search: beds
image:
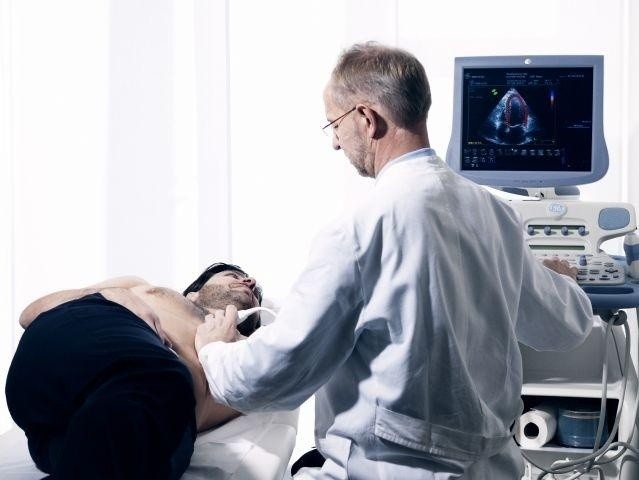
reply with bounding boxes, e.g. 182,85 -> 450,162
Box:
0,410 -> 300,480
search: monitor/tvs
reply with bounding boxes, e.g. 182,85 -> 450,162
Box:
446,55 -> 610,199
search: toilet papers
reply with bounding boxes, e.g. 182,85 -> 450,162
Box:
515,410 -> 557,446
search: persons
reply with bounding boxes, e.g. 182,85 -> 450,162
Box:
193,38 -> 594,480
5,260 -> 264,480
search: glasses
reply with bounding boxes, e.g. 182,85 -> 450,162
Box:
319,108 -> 355,133
